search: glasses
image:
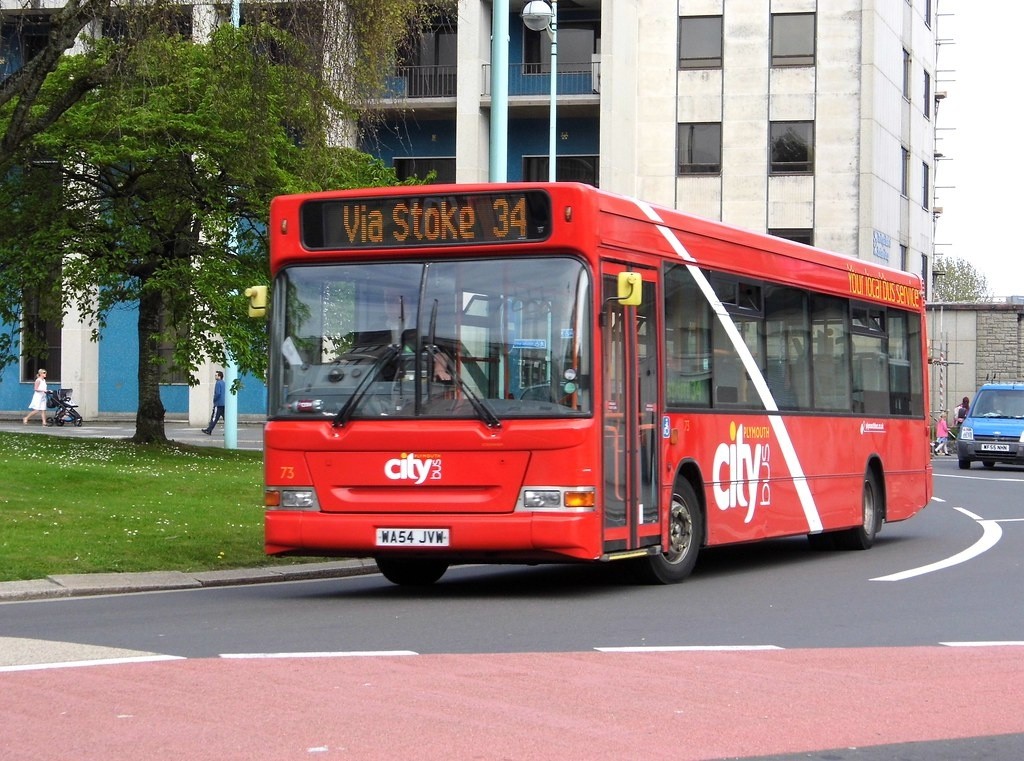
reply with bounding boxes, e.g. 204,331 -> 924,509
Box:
42,372 -> 46,374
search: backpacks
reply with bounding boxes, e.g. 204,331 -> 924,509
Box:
957,407 -> 967,424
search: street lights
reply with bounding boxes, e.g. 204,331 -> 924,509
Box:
521,0 -> 558,184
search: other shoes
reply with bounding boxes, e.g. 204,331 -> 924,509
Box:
202,430 -> 211,436
42,424 -> 48,427
934,450 -> 938,456
23,417 -> 27,425
945,453 -> 951,456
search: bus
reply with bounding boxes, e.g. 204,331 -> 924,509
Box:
242,179 -> 933,586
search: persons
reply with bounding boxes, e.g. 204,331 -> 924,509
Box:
952,396 -> 970,449
354,281 -> 451,384
22,368 -> 52,428
933,412 -> 951,456
201,370 -> 226,435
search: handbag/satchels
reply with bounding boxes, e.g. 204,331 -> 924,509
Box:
47,394 -> 56,408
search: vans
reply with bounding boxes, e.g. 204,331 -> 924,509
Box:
954,382 -> 1024,472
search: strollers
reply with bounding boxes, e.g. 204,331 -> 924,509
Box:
44,388 -> 83,427
